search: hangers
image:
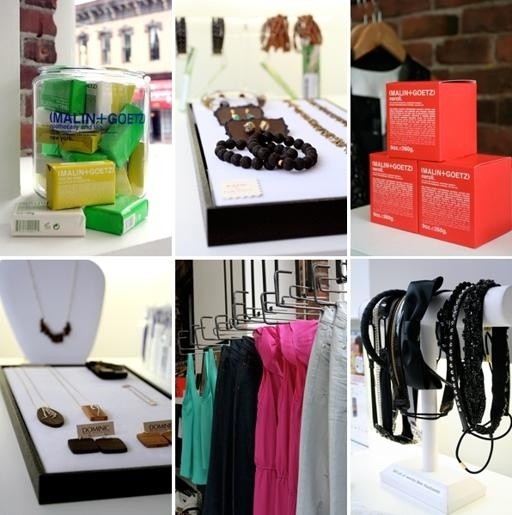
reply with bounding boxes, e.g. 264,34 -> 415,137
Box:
351,0 -> 438,83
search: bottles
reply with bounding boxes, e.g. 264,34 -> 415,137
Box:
30,61 -> 150,198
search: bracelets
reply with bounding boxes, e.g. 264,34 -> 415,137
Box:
246,131 -> 318,170
214,140 -> 298,171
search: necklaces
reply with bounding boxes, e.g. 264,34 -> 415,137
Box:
14,366 -> 64,428
47,366 -> 111,422
28,260 -> 79,345
